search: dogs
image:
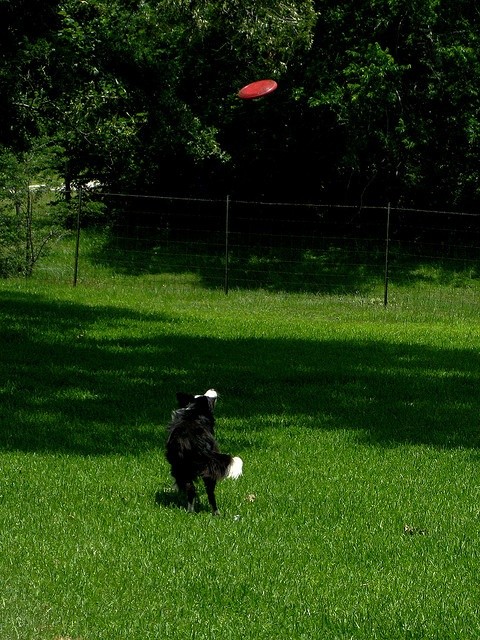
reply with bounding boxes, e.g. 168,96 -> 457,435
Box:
165,389 -> 243,516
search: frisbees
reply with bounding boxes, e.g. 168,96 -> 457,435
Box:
238,80 -> 277,99
